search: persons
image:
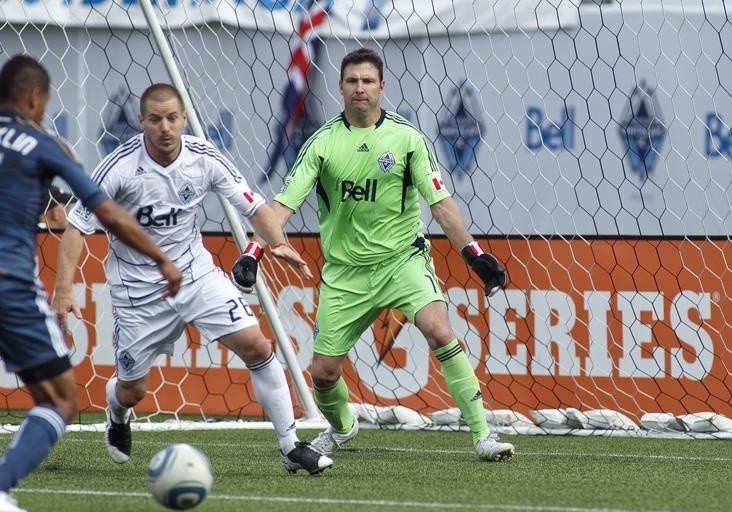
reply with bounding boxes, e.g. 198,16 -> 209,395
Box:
48,82 -> 335,477
228,46 -> 517,463
0,53 -> 184,511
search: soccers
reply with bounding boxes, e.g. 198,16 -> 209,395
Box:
148,442 -> 213,508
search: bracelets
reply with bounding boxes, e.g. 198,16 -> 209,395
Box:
274,242 -> 288,248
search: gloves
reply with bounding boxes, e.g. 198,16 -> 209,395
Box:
460,240 -> 508,297
229,239 -> 264,293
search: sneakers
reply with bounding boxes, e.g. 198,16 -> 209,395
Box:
473,432 -> 517,463
277,440 -> 334,474
102,401 -> 135,465
307,416 -> 360,456
0,491 -> 27,512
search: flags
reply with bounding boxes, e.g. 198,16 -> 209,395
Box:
256,0 -> 329,185
